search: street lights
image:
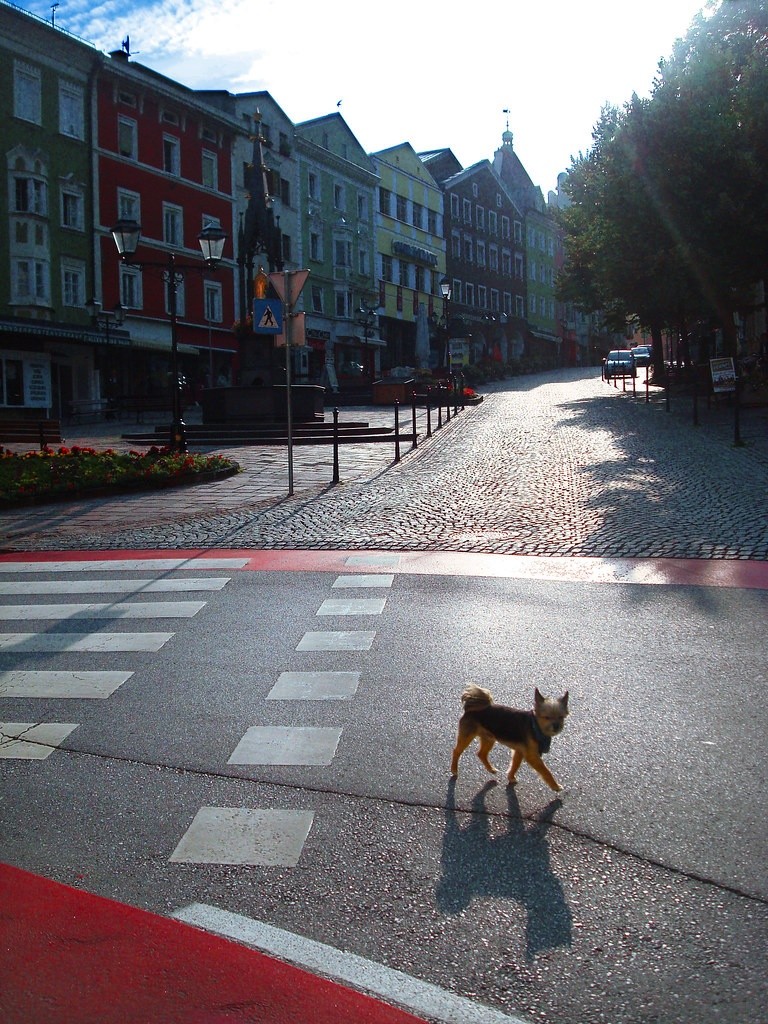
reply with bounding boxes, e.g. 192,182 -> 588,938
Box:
110,210 -> 230,455
440,274 -> 454,387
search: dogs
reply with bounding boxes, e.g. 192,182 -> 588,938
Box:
450,687 -> 570,793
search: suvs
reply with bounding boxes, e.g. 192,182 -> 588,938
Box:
637,345 -> 652,355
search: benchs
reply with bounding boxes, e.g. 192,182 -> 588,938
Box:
64,396 -> 195,425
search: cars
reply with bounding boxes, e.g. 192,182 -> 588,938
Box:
602,350 -> 636,378
631,347 -> 653,367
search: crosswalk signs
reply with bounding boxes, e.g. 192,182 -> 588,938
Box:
252,297 -> 284,334
